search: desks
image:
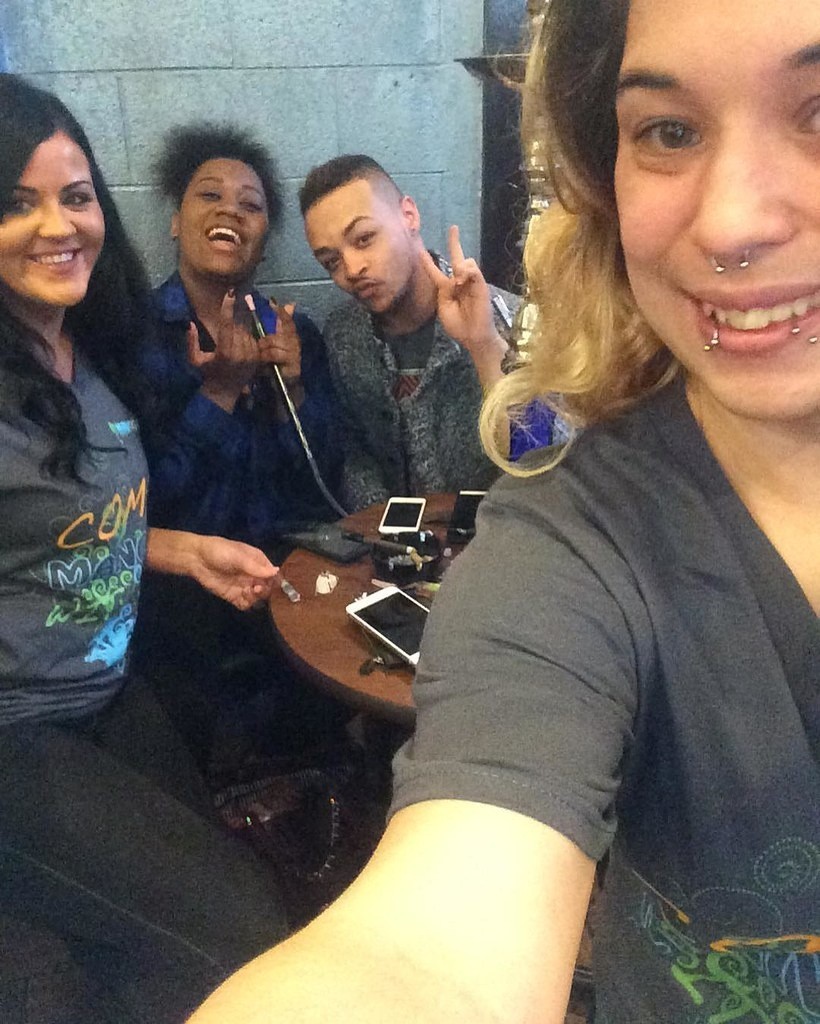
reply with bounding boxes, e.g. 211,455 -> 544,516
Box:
269,493 -> 466,727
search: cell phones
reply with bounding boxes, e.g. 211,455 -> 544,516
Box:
346,586 -> 430,667
378,497 -> 426,534
452,489 -> 488,521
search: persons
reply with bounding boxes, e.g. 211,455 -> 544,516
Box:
180,0 -> 820,1024
0,72 -> 526,1023
